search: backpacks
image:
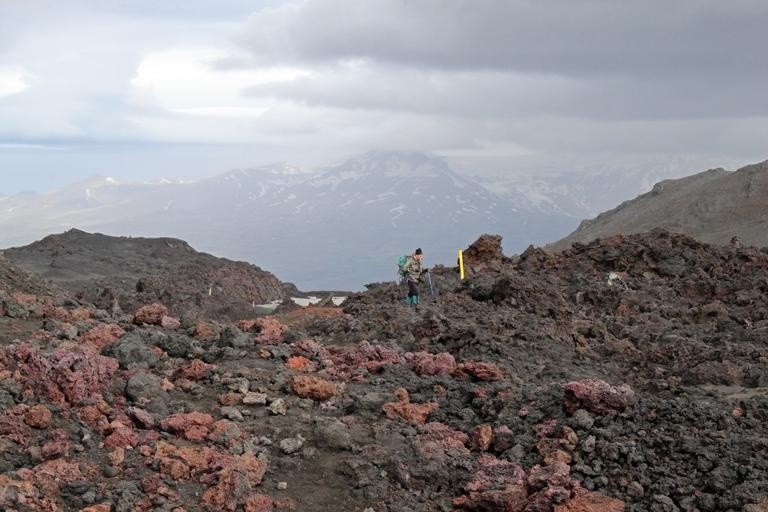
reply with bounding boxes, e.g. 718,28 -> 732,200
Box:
398,255 -> 414,274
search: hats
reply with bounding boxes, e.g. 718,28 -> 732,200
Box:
415,248 -> 422,255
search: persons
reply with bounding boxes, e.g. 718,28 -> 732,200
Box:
397,248 -> 430,306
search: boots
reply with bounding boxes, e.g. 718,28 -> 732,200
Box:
404,295 -> 412,306
412,295 -> 419,305
